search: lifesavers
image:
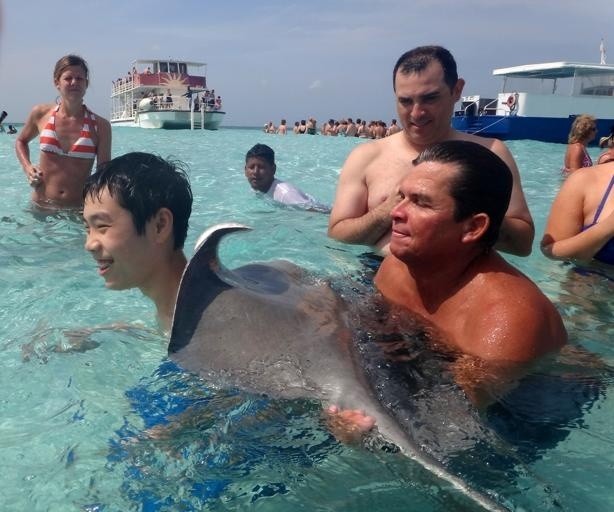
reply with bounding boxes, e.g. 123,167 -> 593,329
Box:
506,96 -> 516,108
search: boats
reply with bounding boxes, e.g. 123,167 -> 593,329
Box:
450,61 -> 614,147
109,59 -> 225,131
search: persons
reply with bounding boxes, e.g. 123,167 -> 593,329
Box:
599,135 -> 614,162
264,117 -> 400,138
58,153 -> 264,478
328,45 -> 535,269
111,68 -> 151,85
15,55 -> 111,219
561,115 -> 599,176
141,90 -> 222,111
245,143 -> 330,214
540,154 -> 613,300
0,124 -> 5,132
7,125 -> 17,134
325,140 -> 582,483
